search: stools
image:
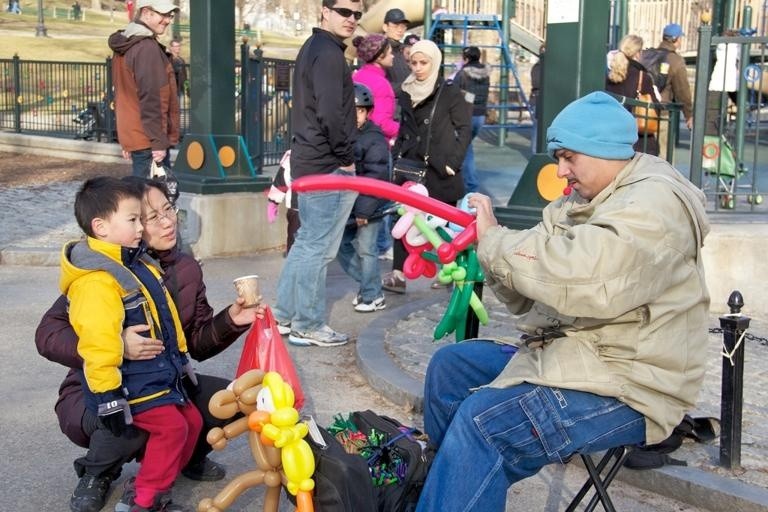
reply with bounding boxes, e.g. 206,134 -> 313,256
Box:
559,432 -> 688,511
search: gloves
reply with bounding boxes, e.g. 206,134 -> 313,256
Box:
97,400 -> 134,438
180,363 -> 200,400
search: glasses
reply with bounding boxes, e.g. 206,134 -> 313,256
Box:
143,204 -> 180,226
328,7 -> 363,22
149,8 -> 174,19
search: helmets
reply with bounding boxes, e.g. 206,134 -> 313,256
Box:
352,83 -> 374,108
663,25 -> 685,40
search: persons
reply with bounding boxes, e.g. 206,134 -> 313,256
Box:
73,2 -> 81,20
605,35 -> 660,158
271,0 -> 359,352
57,175 -> 202,511
33,176 -> 268,512
7,0 -> 22,14
414,88 -> 718,512
708,30 -> 740,106
527,44 -> 543,153
167,40 -> 185,123
108,1 -> 181,182
642,23 -> 696,167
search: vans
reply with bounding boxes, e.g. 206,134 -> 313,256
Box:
677,47 -> 767,107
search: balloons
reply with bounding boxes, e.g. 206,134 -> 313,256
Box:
199,370 -> 315,511
291,162 -> 491,342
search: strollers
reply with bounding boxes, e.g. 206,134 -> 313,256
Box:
73,87 -> 116,144
682,114 -> 761,209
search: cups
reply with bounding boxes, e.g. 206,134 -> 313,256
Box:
233,275 -> 262,308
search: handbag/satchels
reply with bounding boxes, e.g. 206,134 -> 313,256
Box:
390,158 -> 429,188
633,71 -> 658,137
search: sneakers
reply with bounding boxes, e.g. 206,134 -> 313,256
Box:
181,457 -> 226,482
269,319 -> 292,337
380,272 -> 406,294
355,296 -> 387,313
288,324 -> 349,348
69,472 -> 111,511
379,248 -> 396,261
431,280 -> 451,290
352,293 -> 363,306
132,488 -> 195,512
114,476 -> 140,512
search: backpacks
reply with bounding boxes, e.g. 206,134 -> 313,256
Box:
285,405 -> 437,512
641,47 -> 677,93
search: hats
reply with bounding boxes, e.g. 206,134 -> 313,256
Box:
352,34 -> 389,63
545,90 -> 640,160
385,8 -> 410,25
135,1 -> 182,18
402,33 -> 419,48
